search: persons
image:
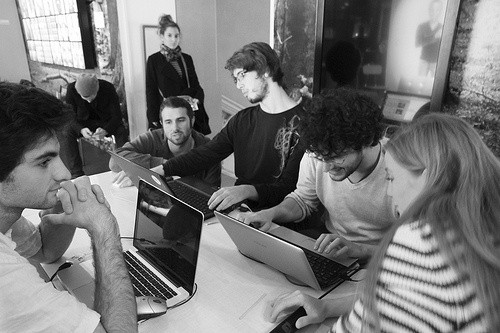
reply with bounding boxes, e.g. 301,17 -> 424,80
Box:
108,97 -> 222,190
229,90 -> 401,260
0,80 -> 139,333
145,14 -> 212,136
64,72 -> 128,179
112,41 -> 310,211
268,113 -> 500,333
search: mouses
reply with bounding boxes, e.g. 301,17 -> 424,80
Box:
250,222 -> 261,229
113,177 -> 132,187
135,296 -> 167,319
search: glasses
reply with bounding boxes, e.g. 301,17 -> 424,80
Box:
233,70 -> 248,83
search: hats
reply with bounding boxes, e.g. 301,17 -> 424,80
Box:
75,73 -> 99,97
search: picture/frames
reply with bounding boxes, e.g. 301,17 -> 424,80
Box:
142,25 -> 163,63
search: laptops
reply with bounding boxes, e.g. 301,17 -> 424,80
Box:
380,91 -> 432,127
106,150 -> 247,221
57,179 -> 204,329
213,210 -> 359,292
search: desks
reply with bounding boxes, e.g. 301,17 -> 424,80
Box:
20,164 -> 367,333
